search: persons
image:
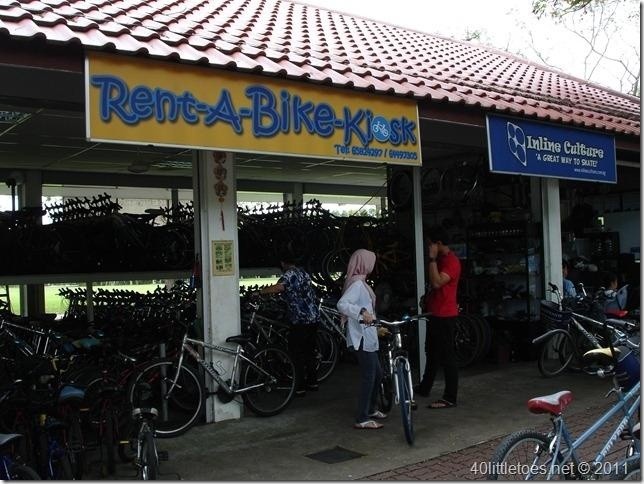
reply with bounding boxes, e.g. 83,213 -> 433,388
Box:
562,261 -> 577,300
414,224 -> 464,409
337,248 -> 389,430
249,249 -> 320,398
595,272 -> 624,312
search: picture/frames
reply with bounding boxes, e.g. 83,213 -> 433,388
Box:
211,239 -> 236,277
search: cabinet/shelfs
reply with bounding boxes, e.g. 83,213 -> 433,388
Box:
464,221 -> 545,363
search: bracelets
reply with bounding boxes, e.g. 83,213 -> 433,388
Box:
257,290 -> 262,296
429,257 -> 437,262
360,306 -> 367,313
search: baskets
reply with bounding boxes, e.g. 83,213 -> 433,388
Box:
540,300 -> 572,332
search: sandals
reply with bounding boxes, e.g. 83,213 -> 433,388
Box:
368,410 -> 387,418
355,420 -> 384,429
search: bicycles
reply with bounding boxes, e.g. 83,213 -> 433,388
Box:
359,312 -> 432,447
445,268 -> 481,370
484,343 -> 640,480
530,277 -> 640,384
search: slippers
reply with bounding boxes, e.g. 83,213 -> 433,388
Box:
430,400 -> 457,408
415,385 -> 432,396
